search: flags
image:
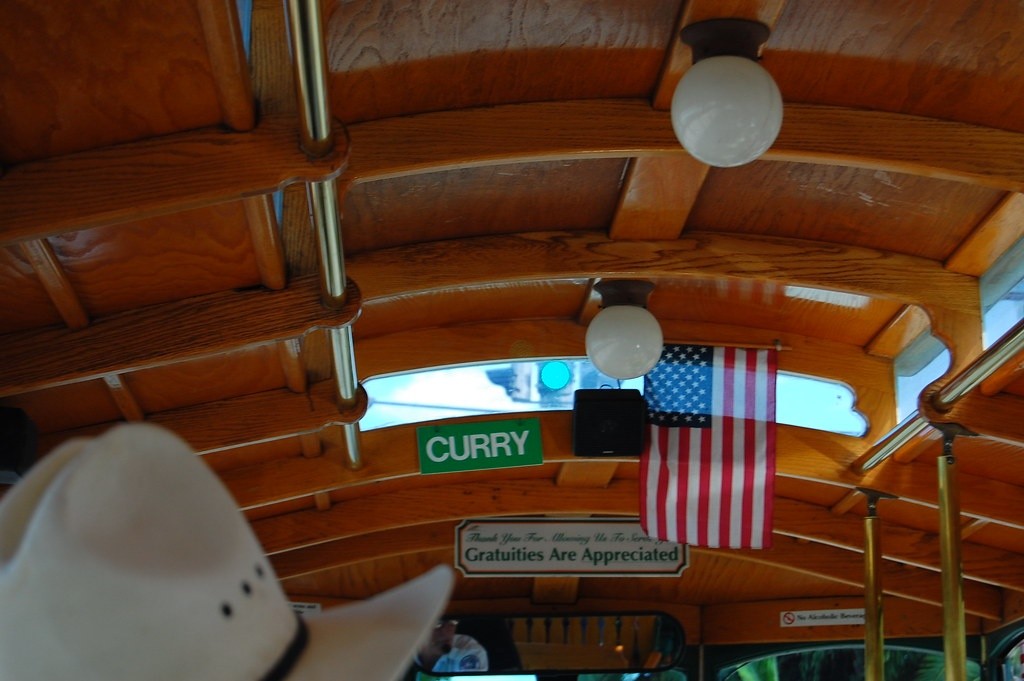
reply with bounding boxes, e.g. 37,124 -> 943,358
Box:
639,344 -> 777,550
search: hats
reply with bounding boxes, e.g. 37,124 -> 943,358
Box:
0,421 -> 453,681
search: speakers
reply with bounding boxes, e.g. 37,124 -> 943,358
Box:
573,390 -> 649,458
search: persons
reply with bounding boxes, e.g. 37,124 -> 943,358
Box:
417,618 -> 487,671
0,425 -> 453,681
